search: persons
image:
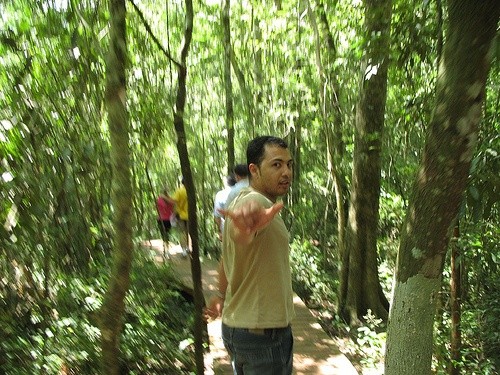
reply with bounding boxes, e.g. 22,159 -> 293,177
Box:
201,136 -> 293,375
213,175 -> 237,242
220,164 -> 250,211
157,190 -> 179,253
160,177 -> 191,258
